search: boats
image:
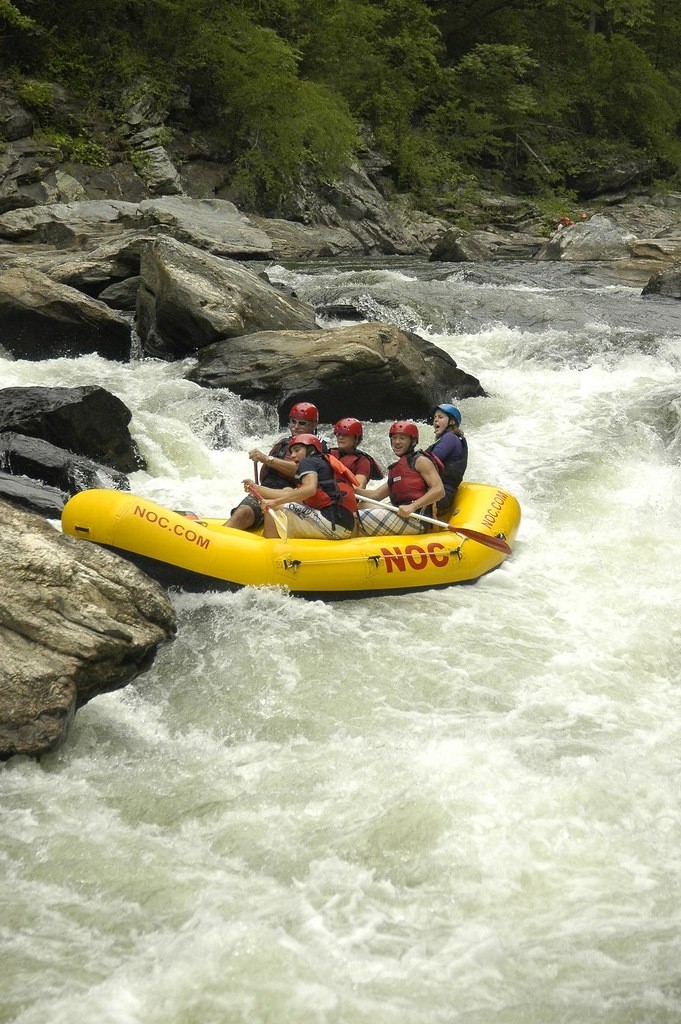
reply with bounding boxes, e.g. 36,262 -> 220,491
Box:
58,481 -> 524,597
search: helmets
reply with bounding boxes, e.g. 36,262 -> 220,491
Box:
289,402 -> 319,423
289,434 -> 322,453
436,403 -> 461,424
334,418 -> 363,437
389,421 -> 419,444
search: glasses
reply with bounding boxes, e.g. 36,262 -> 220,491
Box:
290,418 -> 313,425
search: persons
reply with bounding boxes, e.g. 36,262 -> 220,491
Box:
352,420 -> 446,537
320,416 -> 384,503
241,433 -> 359,541
222,401 -> 331,531
415,403 -> 468,528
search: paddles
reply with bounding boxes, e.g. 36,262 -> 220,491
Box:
353,493 -> 512,555
245,451 -> 289,545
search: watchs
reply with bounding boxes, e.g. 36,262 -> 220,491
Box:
266,455 -> 274,463
